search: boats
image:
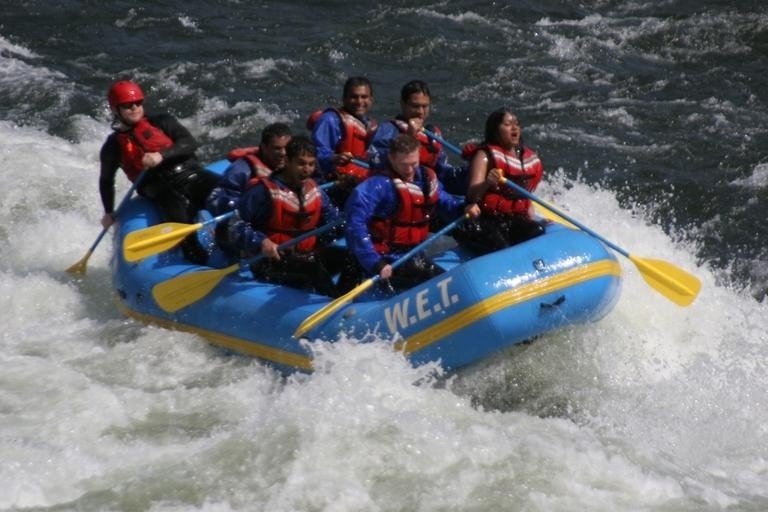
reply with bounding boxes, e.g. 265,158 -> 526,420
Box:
106,144 -> 629,386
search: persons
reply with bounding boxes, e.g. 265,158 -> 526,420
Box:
309,75 -> 376,203
457,106 -> 546,250
228,135 -> 361,297
208,122 -> 292,268
342,132 -> 482,303
100,81 -> 226,264
367,81 -> 473,195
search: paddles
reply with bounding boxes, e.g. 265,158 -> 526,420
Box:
66,167 -> 149,276
124,179 -> 341,263
422,127 -> 580,230
293,212 -> 471,340
152,219 -> 344,315
500,176 -> 703,308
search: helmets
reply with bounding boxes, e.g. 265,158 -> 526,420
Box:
106,79 -> 144,107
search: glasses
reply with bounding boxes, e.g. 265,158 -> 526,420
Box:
121,101 -> 143,109
